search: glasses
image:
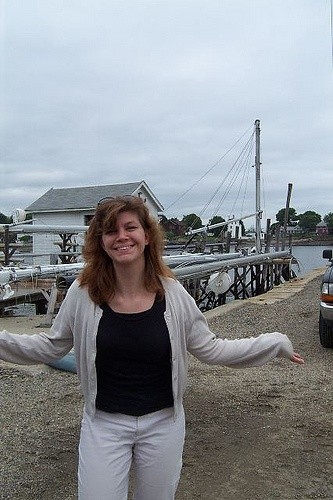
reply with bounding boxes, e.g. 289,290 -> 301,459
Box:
97,195 -> 137,206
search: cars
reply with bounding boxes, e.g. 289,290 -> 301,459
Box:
319,249 -> 333,348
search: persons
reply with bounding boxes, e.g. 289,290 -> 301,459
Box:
0,192 -> 306,500
